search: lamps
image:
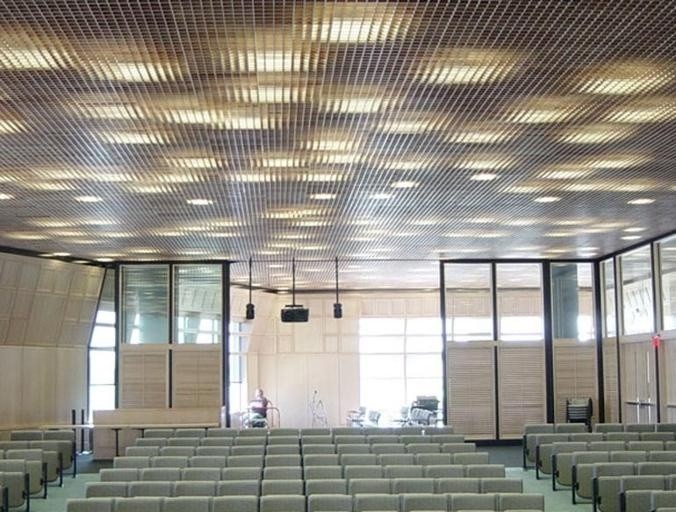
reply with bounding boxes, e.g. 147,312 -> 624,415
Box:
247,257 -> 254,319
334,257 -> 341,318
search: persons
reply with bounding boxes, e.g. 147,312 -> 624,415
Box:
251,388 -> 268,427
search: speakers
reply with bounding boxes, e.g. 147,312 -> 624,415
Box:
333,303 -> 342,318
281,309 -> 309,322
246,304 -> 254,320
